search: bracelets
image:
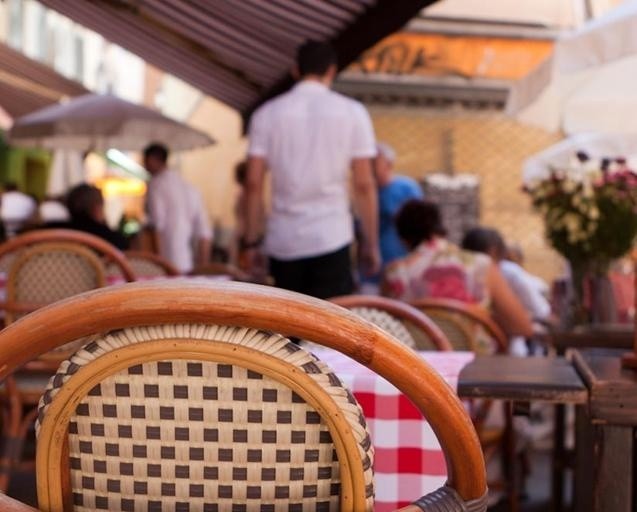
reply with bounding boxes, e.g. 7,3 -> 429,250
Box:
238,233 -> 263,251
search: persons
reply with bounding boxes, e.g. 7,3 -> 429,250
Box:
505,241 -> 551,357
142,140 -> 214,277
353,141 -> 424,298
459,228 -> 561,358
228,162 -> 265,272
35,182 -> 130,253
236,38 -> 381,304
379,198 -> 533,428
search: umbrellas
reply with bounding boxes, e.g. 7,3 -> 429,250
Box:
520,132 -> 637,186
501,0 -> 637,132
9,82 -> 215,162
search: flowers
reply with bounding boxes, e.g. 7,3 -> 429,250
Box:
517,150 -> 637,280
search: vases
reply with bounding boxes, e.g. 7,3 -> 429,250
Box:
564,271 -> 619,327
